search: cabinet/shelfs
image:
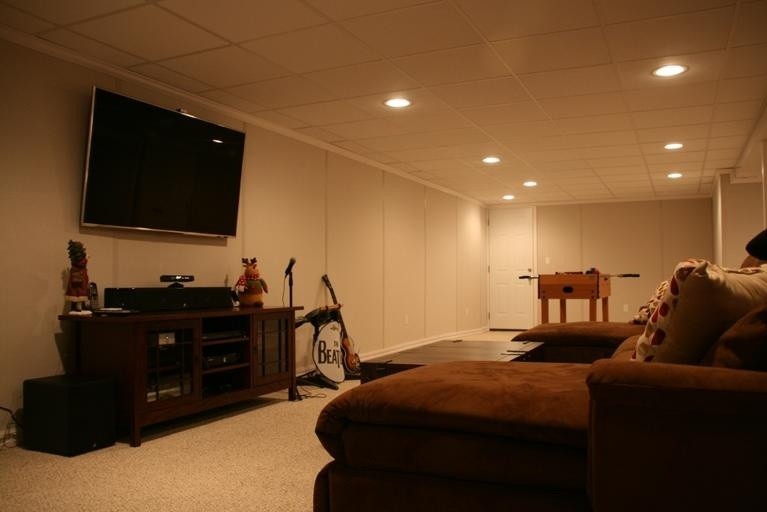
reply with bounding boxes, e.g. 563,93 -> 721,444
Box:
57,304 -> 303,449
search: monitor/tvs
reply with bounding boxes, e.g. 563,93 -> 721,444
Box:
81,86 -> 246,238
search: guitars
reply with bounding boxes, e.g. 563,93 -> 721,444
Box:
322,274 -> 361,374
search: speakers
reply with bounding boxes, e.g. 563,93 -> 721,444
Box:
104,287 -> 233,315
22,374 -> 116,457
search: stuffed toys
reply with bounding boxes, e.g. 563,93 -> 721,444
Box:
232,256 -> 270,309
63,239 -> 91,312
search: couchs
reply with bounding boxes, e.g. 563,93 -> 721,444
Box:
303,244 -> 767,511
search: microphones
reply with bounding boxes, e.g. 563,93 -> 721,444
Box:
285,258 -> 297,276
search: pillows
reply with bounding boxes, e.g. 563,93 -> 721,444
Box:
621,255 -> 765,369
629,271 -> 672,324
